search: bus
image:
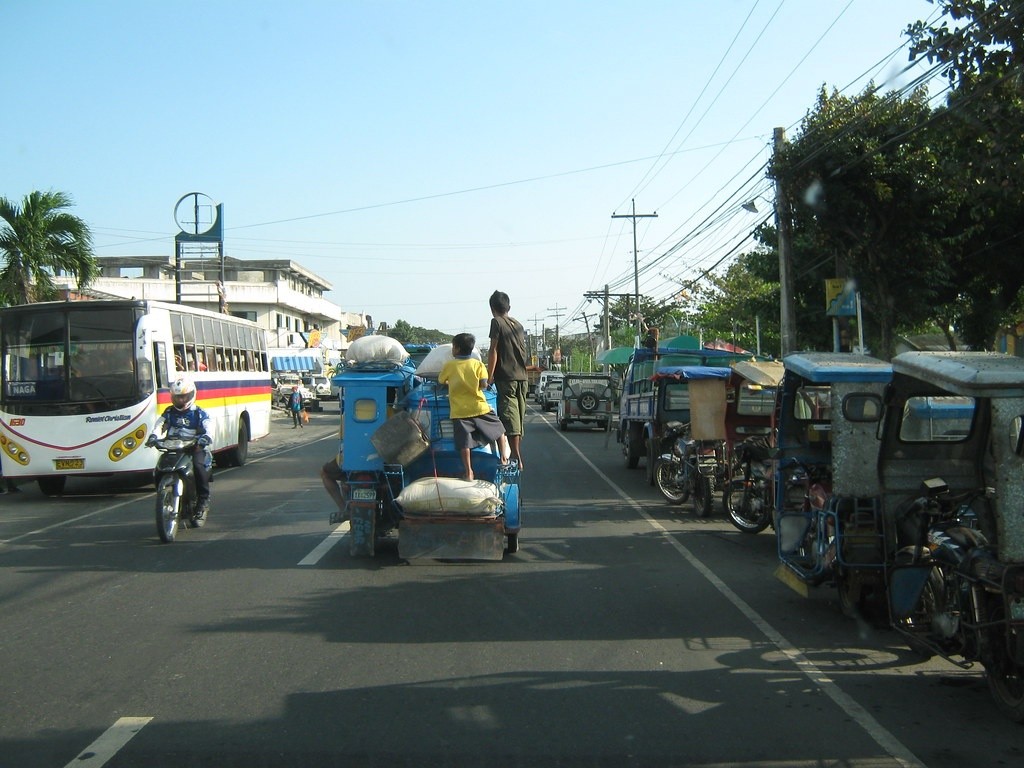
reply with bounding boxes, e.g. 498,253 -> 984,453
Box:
0,292 -> 272,496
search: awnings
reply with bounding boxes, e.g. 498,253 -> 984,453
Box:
272,356 -> 316,371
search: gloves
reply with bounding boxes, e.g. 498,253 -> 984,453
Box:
146,434 -> 158,446
197,435 -> 212,445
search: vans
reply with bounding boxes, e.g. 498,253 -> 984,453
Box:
534,370 -> 564,413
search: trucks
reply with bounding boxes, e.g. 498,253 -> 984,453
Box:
608,344 -> 744,469
271,369 -> 320,413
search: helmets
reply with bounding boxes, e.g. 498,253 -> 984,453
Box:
170,376 -> 196,411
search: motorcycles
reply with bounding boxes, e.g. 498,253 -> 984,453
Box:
651,356 -> 809,518
722,348 -> 976,629
329,342 -> 523,570
841,350 -> 1023,725
143,426 -> 214,544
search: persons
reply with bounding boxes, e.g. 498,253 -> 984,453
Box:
321,390 -> 395,522
487,290 -> 529,471
438,333 -> 511,482
148,376 -> 211,528
287,386 -> 305,429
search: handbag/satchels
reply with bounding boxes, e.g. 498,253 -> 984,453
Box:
303,410 -> 309,423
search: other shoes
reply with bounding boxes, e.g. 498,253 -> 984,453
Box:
300,423 -> 303,428
496,458 -> 518,470
194,498 -> 206,519
292,426 -> 297,429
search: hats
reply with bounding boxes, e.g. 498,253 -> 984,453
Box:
292,386 -> 298,391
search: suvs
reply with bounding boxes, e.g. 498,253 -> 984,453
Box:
557,372 -> 615,433
311,372 -> 332,401
